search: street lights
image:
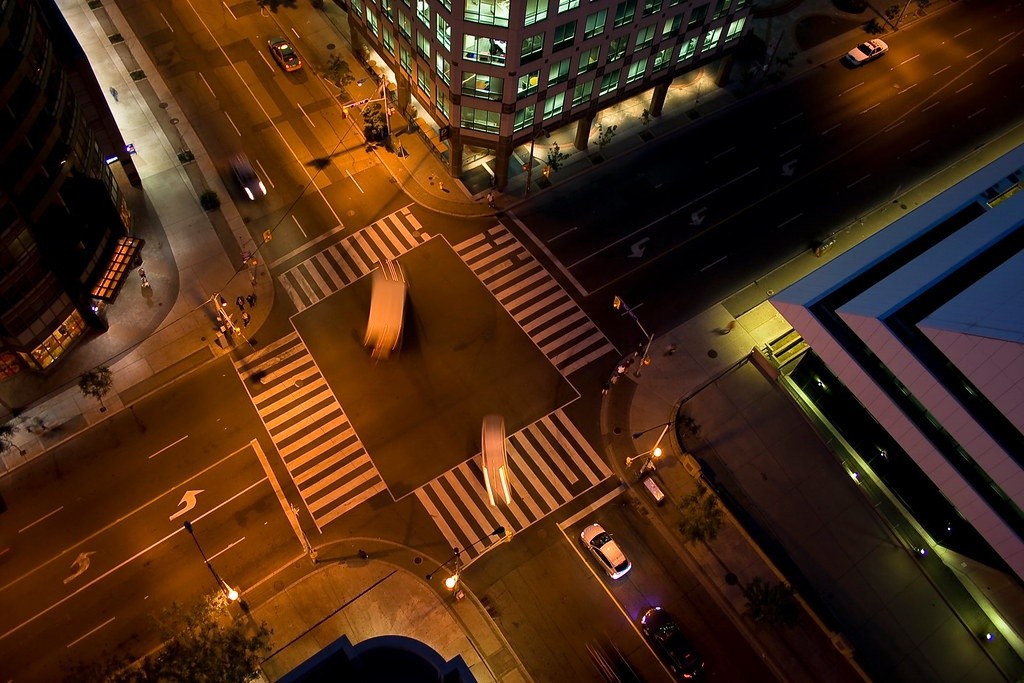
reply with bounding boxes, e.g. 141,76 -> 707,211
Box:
426,525 -> 508,595
523,128 -> 551,198
184,520 -> 229,601
356,72 -> 392,147
632,422 -> 672,479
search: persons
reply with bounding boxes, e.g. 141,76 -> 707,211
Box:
138,268 -> 148,287
487,192 -> 495,208
237,294 -> 254,328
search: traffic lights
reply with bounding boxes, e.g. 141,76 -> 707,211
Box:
342,106 -> 348,120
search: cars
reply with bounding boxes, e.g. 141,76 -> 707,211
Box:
581,523 -> 632,579
267,35 -> 302,73
228,153 -> 267,202
640,606 -> 706,683
846,38 -> 889,66
